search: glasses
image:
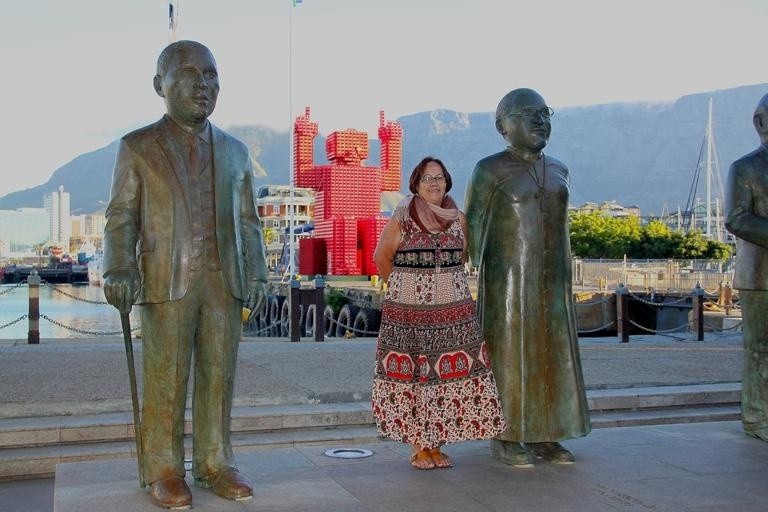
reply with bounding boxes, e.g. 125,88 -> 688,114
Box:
419,173 -> 446,184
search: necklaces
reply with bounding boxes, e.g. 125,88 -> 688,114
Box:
510,147 -> 551,199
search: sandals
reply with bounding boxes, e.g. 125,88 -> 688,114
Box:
409,449 -> 435,470
430,452 -> 453,468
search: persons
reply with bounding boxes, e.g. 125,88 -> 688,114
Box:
373,156 -> 504,470
102,41 -> 267,508
724,93 -> 768,442
465,88 -> 591,466
